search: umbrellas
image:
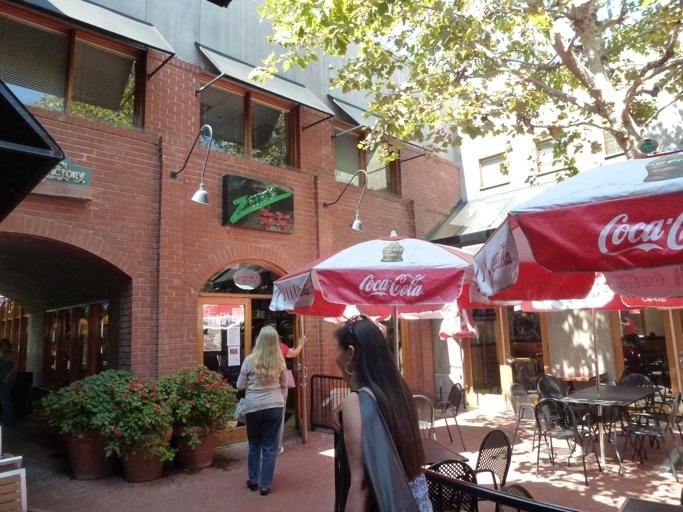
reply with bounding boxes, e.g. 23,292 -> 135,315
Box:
268,231 -> 483,373
471,153 -> 683,300
436,289 -> 479,388
468,274 -> 682,469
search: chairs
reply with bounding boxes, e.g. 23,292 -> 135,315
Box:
0,455 -> 28,511
412,371 -> 683,512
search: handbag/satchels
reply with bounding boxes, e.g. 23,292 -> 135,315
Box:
233,398 -> 248,425
287,370 -> 297,388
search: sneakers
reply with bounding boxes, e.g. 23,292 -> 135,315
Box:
247,481 -> 259,491
260,487 -> 270,495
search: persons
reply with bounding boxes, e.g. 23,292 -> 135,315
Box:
514,315 -> 522,335
260,335 -> 308,459
236,326 -> 288,496
621,314 -> 640,349
0,338 -> 18,424
332,315 -> 433,511
523,320 -> 540,341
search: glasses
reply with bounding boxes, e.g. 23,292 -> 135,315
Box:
345,314 -> 369,354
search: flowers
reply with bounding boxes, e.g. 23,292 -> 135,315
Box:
42,369 -> 137,438
156,364 -> 238,429
94,379 -> 174,443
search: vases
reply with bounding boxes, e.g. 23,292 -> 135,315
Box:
116,428 -> 174,482
66,435 -> 110,479
169,426 -> 212,471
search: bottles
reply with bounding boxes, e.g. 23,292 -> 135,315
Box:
380,241 -> 404,263
644,152 -> 683,183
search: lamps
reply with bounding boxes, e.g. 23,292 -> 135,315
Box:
323,169 -> 368,231
170,124 -> 212,205
